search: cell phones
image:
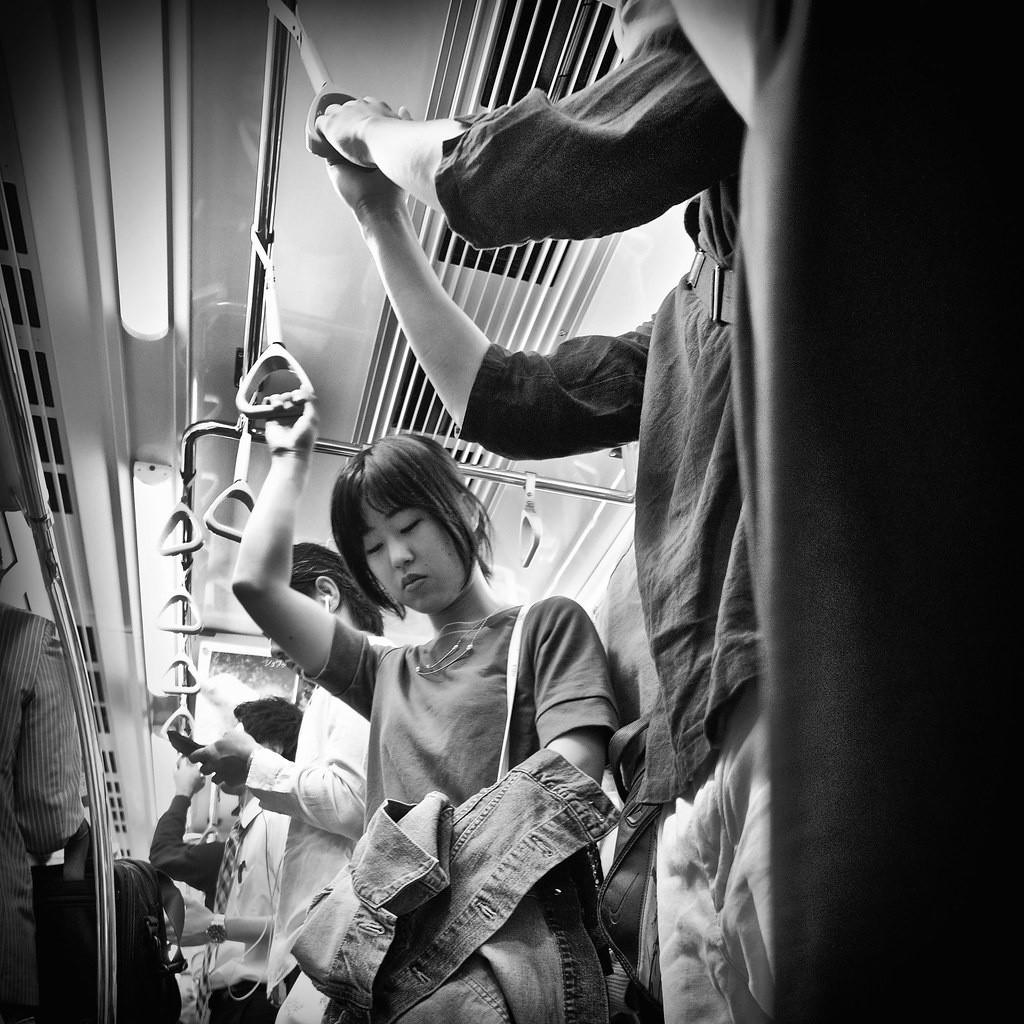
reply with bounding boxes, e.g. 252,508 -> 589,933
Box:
167,731 -> 204,756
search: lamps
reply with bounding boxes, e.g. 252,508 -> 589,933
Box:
92,0 -> 170,344
152,725 -> 185,830
132,459 -> 180,698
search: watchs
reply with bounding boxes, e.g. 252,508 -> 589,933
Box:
205,914 -> 228,944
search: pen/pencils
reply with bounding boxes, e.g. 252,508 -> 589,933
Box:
239,860 -> 246,884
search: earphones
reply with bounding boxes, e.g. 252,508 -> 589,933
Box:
323,594 -> 331,611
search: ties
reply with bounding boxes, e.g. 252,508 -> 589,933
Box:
216,821 -> 243,914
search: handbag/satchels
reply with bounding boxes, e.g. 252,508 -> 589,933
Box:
31,854 -> 189,1024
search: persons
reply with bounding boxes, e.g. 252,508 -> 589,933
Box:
146,541 -> 384,1024
230,384 -> 612,976
599,0 -> 1023,1023
318,0 -> 772,1023
0,603 -> 82,1024
